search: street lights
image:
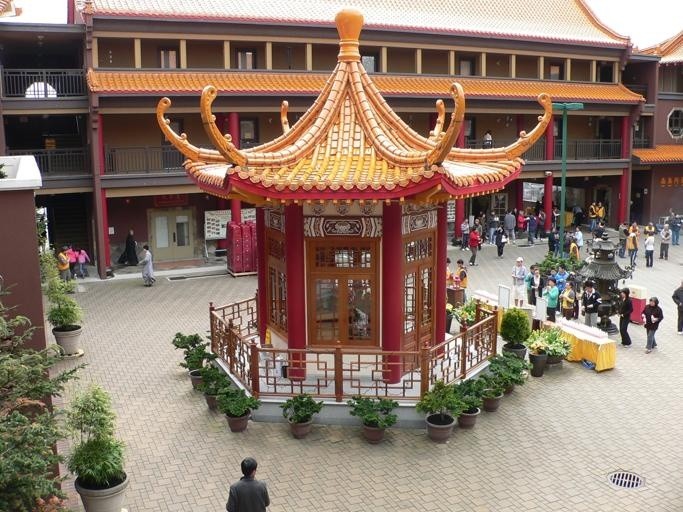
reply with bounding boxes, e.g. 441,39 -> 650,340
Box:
548,100 -> 584,261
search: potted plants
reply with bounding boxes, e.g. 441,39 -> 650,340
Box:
416,378 -> 470,443
529,331 -> 554,377
189,350 -> 218,388
216,388 -> 263,433
197,363 -> 231,410
453,378 -> 486,430
66,388 -> 129,512
488,352 -> 533,395
446,297 -> 572,366
534,250 -> 589,283
478,372 -> 507,412
347,394 -> 400,441
281,392 -> 324,440
43,275 -> 83,355
172,333 -> 211,372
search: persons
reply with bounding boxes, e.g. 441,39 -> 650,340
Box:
57,245 -> 91,282
482,132 -> 492,149
118,229 -> 138,265
446,196 -> 683,354
136,246 -> 155,286
226,457 -> 270,512
